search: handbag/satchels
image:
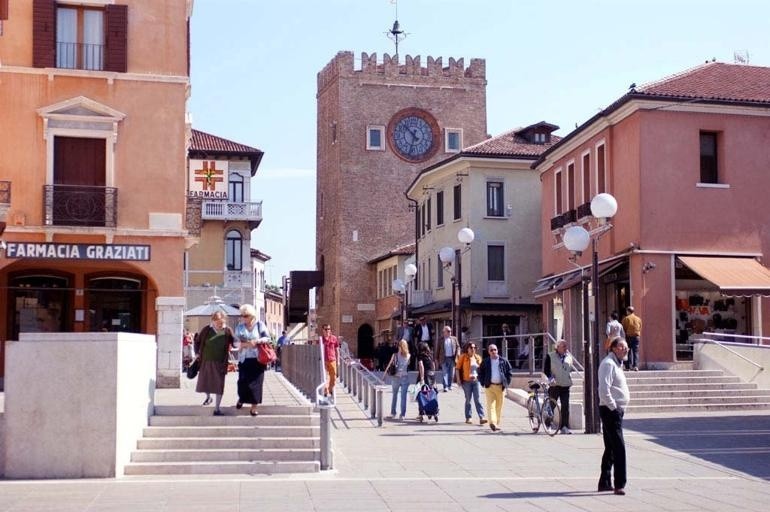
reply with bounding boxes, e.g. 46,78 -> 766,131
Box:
187,358 -> 198,378
387,364 -> 396,376
256,342 -> 277,363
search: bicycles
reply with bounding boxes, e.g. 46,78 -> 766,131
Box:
527,380 -> 562,436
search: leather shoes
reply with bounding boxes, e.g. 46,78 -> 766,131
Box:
614,488 -> 625,495
214,410 -> 223,415
250,409 -> 257,416
236,402 -> 242,409
203,398 -> 212,405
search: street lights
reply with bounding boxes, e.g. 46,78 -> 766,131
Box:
563,192 -> 619,436
439,228 -> 476,346
391,263 -> 418,328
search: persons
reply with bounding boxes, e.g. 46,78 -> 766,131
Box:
233,304 -> 271,416
597,337 -> 631,496
184,326 -> 194,362
375,315 -> 460,420
338,336 -> 349,359
455,341 -> 488,424
316,324 -> 340,397
621,305 -> 641,370
518,330 -> 543,368
495,323 -> 519,348
477,343 -> 512,431
194,308 -> 236,415
276,330 -> 287,346
606,311 -> 626,350
544,339 -> 573,435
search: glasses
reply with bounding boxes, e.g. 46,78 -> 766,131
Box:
470,347 -> 477,349
490,349 -> 496,352
323,328 -> 330,331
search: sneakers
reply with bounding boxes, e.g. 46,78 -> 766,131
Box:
562,427 -> 571,434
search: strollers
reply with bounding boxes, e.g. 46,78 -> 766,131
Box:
416,384 -> 439,423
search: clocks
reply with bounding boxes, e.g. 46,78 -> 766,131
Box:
387,107 -> 442,163
